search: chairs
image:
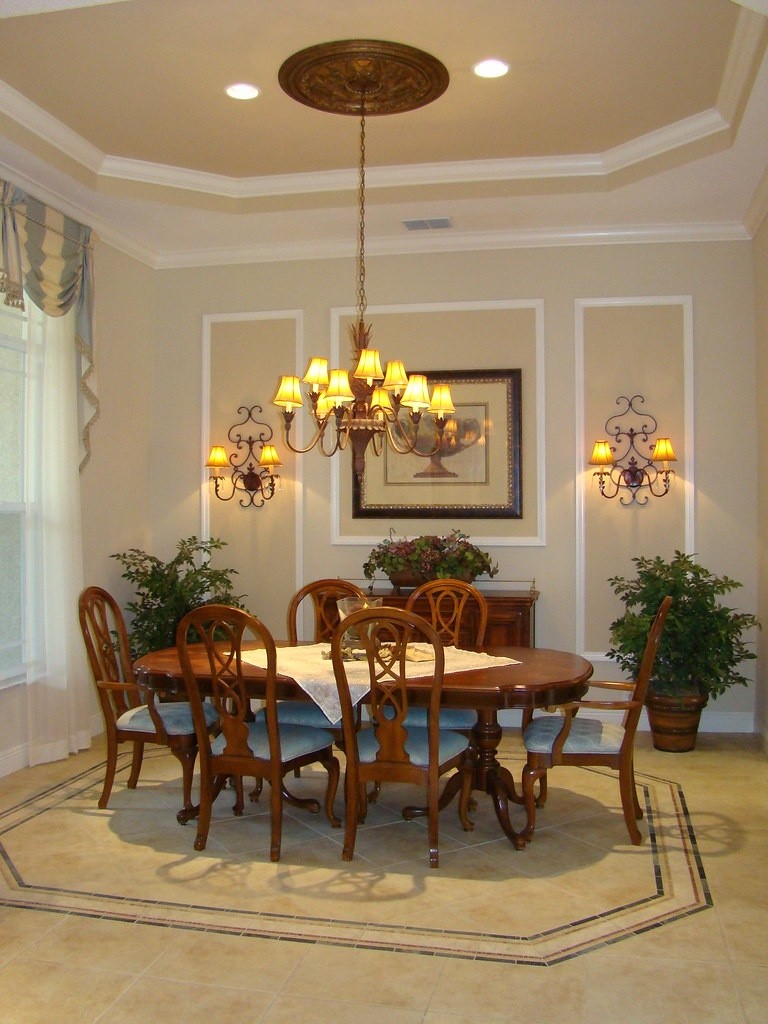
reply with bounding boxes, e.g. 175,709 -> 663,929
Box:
174,604 -> 340,863
371,578 -> 488,811
78,586 -> 227,809
521,597 -> 672,846
254,579 -> 381,804
331,606 -> 474,868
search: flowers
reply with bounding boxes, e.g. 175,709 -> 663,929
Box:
363,528 -> 499,589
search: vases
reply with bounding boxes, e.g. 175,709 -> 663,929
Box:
389,565 -> 477,590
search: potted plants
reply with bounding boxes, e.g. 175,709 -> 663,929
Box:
604,550 -> 762,752
102,535 -> 258,703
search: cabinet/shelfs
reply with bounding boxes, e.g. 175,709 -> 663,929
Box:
318,590 -> 541,649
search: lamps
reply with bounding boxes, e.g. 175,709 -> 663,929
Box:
588,395 -> 678,505
271,38 -> 456,456
205,405 -> 284,508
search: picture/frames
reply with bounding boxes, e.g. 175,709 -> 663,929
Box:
352,368 -> 523,519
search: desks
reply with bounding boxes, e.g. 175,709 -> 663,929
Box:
132,639 -> 594,850
